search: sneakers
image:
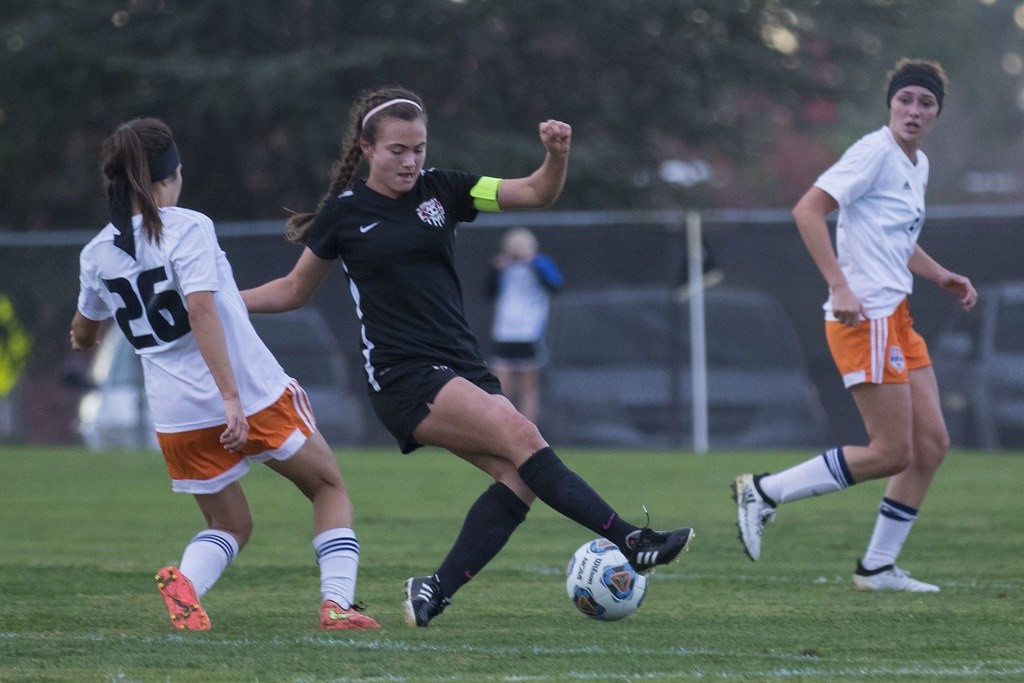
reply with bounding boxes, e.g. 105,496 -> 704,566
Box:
154,566 -> 212,632
320,600 -> 382,630
629,505 -> 695,577
730,473 -> 778,563
852,558 -> 941,593
402,576 -> 452,627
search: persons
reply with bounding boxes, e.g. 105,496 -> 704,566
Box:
733,60 -> 977,593
235,87 -> 694,629
70,117 -> 378,632
485,225 -> 561,429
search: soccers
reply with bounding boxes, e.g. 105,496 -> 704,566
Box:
566,536 -> 648,622
